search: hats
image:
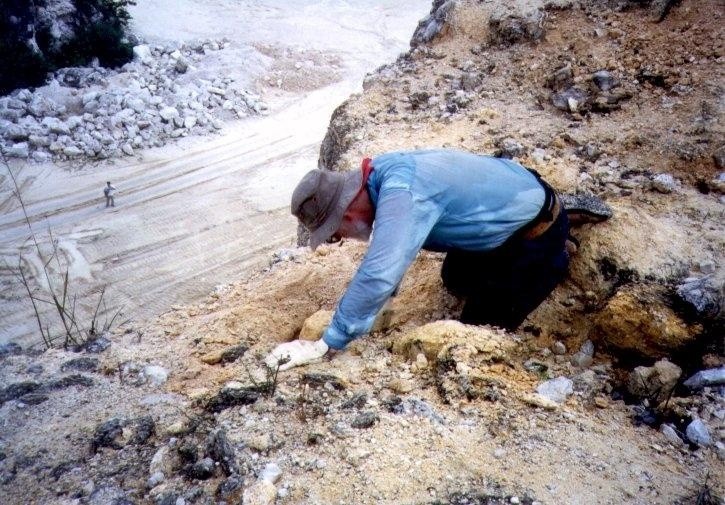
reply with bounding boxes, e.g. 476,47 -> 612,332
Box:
291,167 -> 363,252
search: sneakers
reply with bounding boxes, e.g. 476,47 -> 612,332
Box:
560,192 -> 612,223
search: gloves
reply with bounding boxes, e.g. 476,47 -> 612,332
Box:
265,338 -> 329,371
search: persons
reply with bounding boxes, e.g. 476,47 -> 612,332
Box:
104,182 -> 116,207
265,148 -> 612,373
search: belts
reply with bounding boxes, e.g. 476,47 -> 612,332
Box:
524,192 -> 560,239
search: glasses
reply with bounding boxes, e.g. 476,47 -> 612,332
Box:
325,234 -> 339,243
